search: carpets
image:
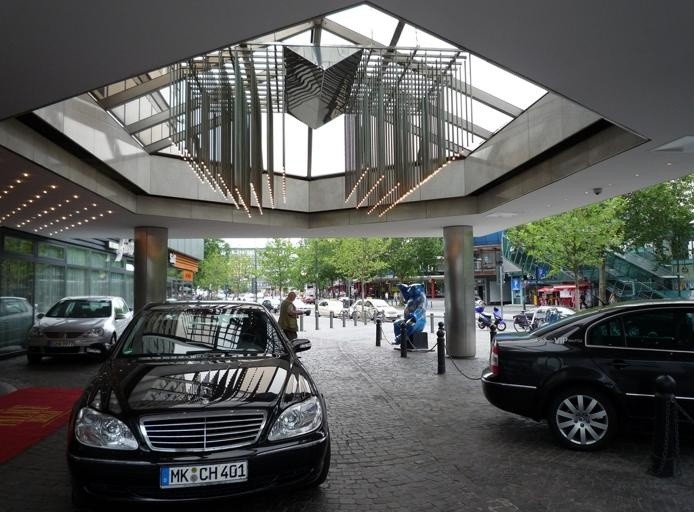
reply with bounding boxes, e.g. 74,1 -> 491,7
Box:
0,385 -> 86,463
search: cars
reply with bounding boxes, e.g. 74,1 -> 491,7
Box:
481,298 -> 694,453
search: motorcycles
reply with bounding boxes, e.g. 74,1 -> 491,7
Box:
474,305 -> 506,331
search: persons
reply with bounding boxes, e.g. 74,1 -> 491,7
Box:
579,292 -> 588,309
607,293 -> 617,304
278,292 -> 306,358
